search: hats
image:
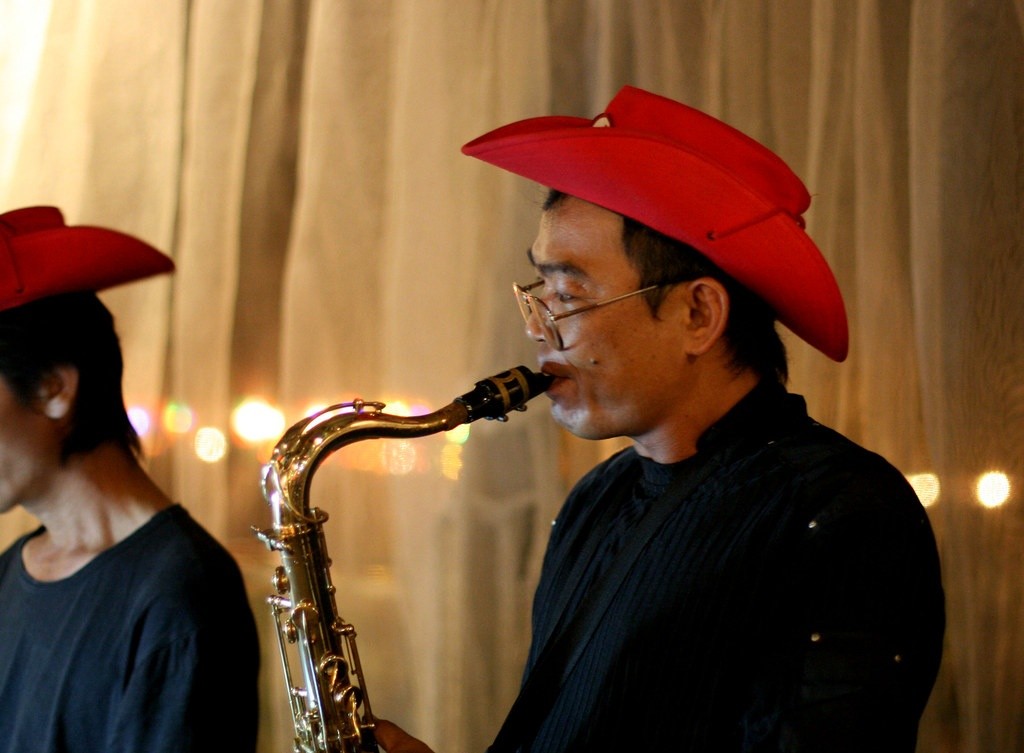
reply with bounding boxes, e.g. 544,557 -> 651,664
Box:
461,84 -> 850,363
0,206 -> 174,313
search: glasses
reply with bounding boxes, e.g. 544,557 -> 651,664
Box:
513,278 -> 704,350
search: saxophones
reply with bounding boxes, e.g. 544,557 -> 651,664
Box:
251,362 -> 569,753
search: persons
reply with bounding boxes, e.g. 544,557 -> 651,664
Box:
0,207 -> 262,753
371,86 -> 945,752
